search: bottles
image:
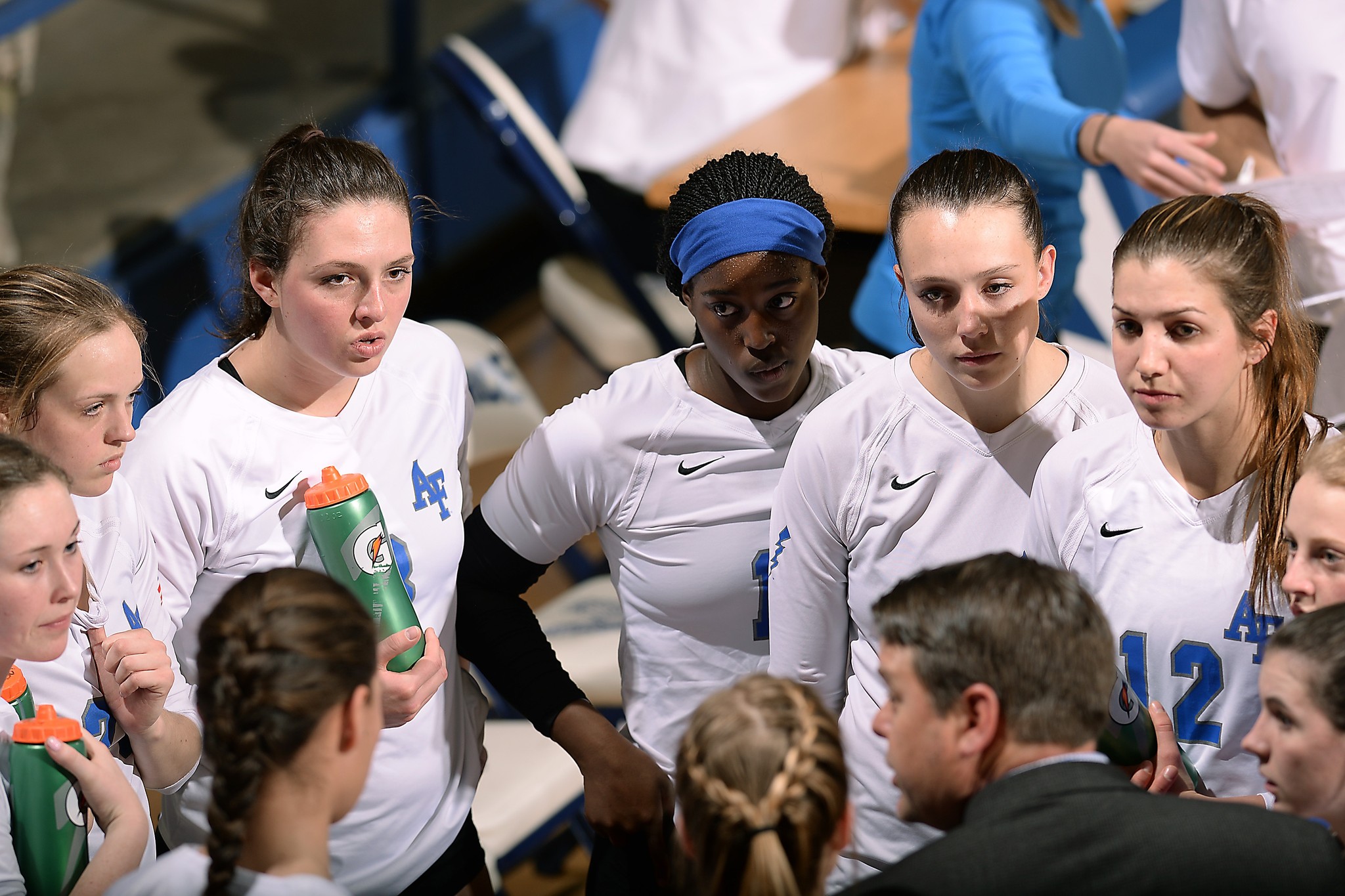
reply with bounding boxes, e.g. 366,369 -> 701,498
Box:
10,704 -> 90,896
304,466 -> 427,673
1,662 -> 36,719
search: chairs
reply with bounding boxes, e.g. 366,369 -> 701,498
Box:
82,110 -> 623,858
434,0 -> 681,351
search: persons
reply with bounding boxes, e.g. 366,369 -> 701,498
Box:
0,1 -> 1345,896
766,149 -> 1129,895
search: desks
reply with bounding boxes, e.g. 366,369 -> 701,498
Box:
648,0 -> 1126,231
